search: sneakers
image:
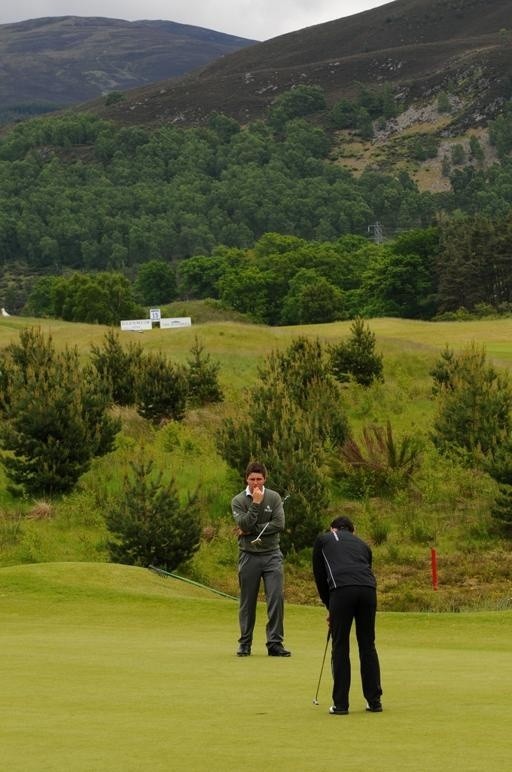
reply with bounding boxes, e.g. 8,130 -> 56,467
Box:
237,647 -> 252,655
329,706 -> 349,715
366,703 -> 383,711
271,647 -> 291,657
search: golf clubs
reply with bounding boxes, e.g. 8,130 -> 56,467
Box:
313,628 -> 331,705
251,494 -> 290,546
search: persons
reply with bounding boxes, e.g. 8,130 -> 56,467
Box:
310,516 -> 383,714
230,463 -> 292,656
